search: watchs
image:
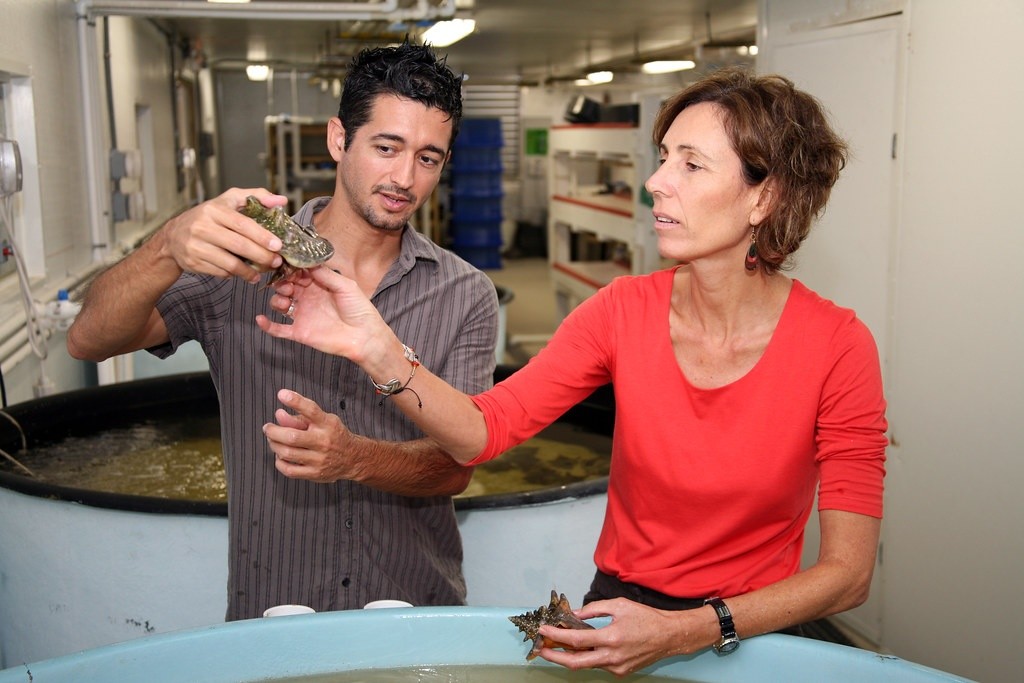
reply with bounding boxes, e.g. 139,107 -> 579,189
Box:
705,597 -> 741,657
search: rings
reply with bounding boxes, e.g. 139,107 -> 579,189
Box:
282,298 -> 295,318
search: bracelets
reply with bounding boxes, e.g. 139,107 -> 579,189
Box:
370,344 -> 423,410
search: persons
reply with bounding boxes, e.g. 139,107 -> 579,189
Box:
256,73 -> 891,682
64,35 -> 500,620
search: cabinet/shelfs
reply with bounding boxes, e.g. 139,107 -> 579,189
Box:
547,95 -> 685,330
